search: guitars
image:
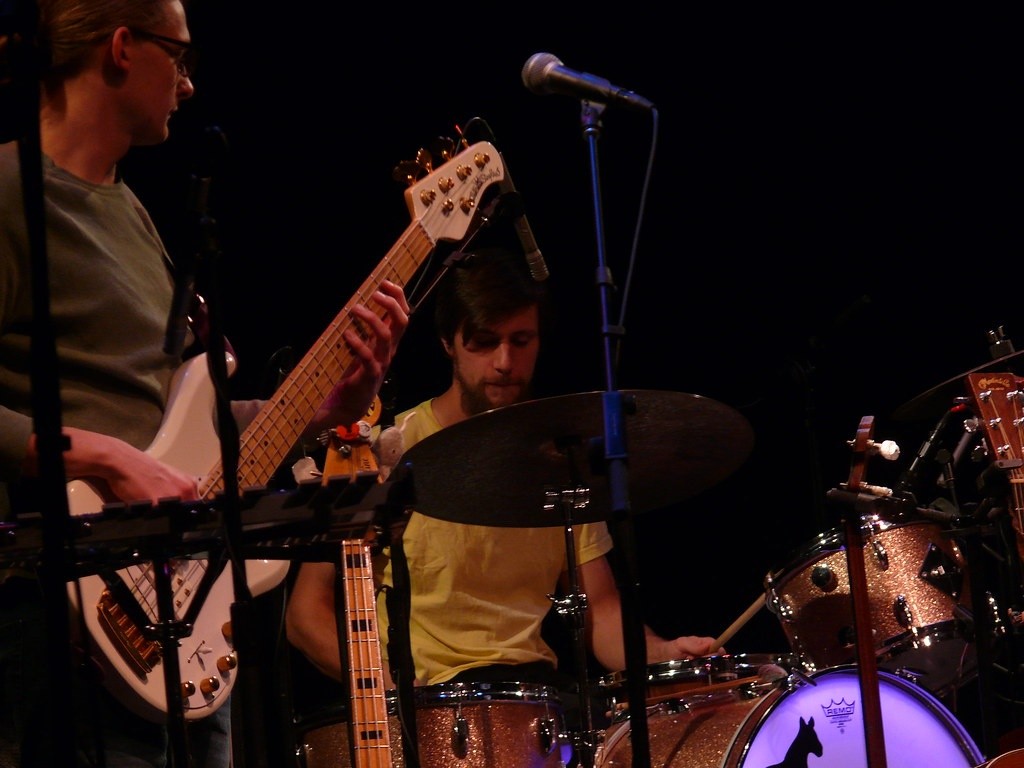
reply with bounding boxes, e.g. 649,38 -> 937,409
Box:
966,366 -> 1024,768
52,121 -> 512,721
293,417 -> 396,768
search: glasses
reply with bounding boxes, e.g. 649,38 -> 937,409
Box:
131,28 -> 198,77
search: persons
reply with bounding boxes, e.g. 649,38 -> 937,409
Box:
0,0 -> 410,767
284,262 -> 727,701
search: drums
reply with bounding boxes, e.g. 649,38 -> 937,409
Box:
766,511 -> 976,698
302,680 -> 580,768
589,650 -> 791,724
593,663 -> 984,767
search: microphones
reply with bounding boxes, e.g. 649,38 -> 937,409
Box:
477,116 -> 549,280
521,52 -> 655,113
936,418 -> 979,489
896,416 -> 948,492
164,124 -> 219,358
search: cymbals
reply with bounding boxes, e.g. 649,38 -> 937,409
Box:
393,386 -> 756,527
